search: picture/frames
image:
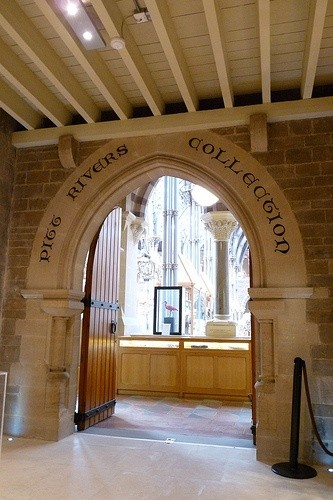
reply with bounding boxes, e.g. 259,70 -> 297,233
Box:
151,285 -> 184,337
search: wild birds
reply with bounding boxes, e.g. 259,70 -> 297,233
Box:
162,301 -> 179,317
